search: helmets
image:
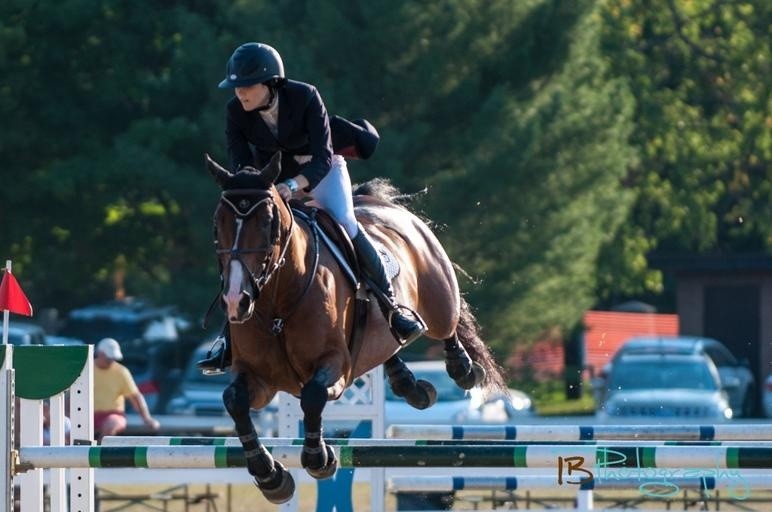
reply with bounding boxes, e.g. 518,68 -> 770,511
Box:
217,42 -> 285,90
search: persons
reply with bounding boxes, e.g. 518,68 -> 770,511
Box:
195,42 -> 424,369
94,338 -> 160,445
43,399 -> 71,445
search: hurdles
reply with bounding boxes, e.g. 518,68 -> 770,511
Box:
0,345 -> 772,512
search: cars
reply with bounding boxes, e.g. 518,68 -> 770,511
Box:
588,335 -> 758,424
0,297 -> 531,437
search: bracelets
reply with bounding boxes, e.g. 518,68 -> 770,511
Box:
284,178 -> 298,192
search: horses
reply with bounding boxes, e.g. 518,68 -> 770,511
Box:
205,150 -> 513,505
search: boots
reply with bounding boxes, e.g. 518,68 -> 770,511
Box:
350,221 -> 424,340
194,322 -> 232,370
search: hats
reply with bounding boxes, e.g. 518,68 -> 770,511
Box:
97,337 -> 124,361
